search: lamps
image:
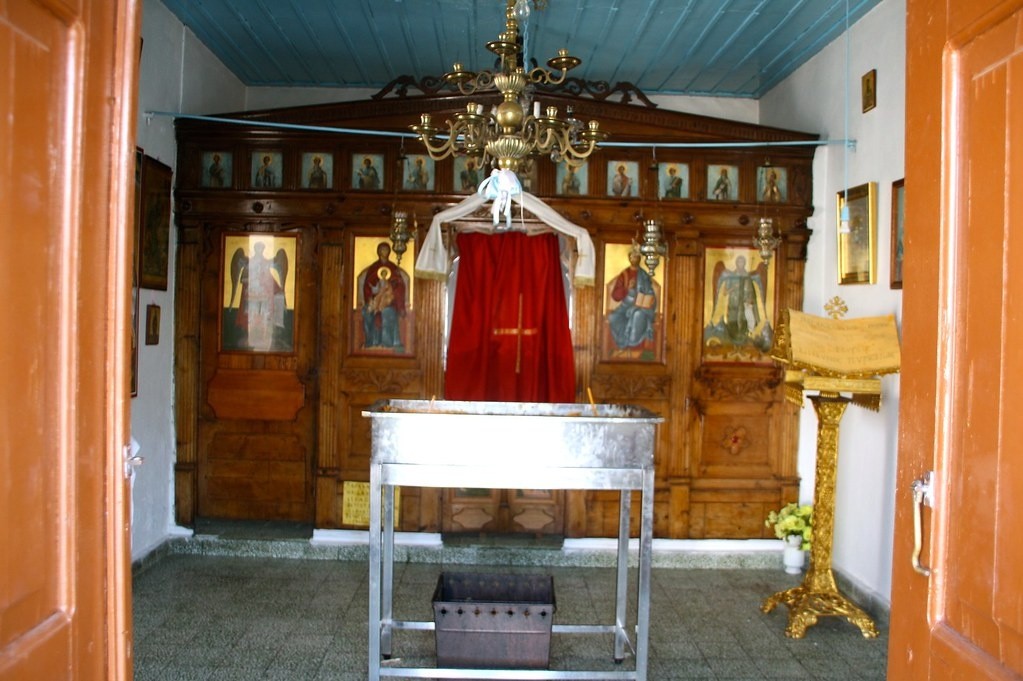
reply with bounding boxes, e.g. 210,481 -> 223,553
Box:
409,0 -> 611,172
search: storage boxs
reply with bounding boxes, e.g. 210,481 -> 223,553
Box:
431,572 -> 557,670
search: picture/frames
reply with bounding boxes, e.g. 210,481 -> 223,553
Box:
145,304 -> 161,345
139,153 -> 172,291
837,181 -> 876,285
889,178 -> 904,290
130,146 -> 144,398
862,69 -> 876,113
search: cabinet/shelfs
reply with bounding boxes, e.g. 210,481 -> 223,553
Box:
171,56 -> 822,539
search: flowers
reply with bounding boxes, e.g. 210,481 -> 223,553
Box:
765,502 -> 813,550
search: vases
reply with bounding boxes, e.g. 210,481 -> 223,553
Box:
780,535 -> 804,575
